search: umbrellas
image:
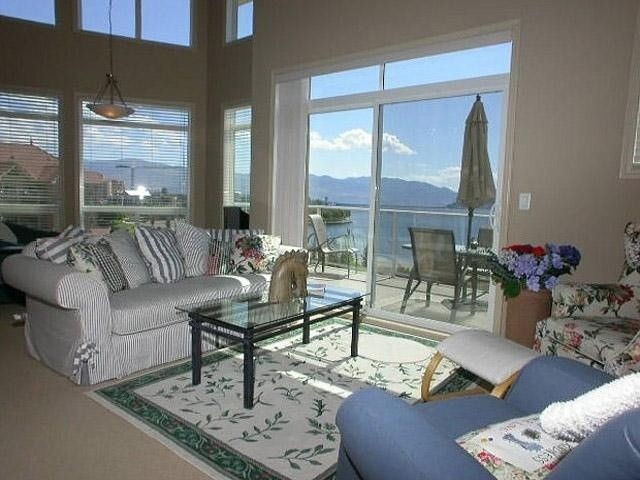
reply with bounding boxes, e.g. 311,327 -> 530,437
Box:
456,95 -> 496,298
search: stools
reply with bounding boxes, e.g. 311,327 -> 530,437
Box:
419,327 -> 544,401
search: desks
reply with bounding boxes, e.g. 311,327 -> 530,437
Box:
399,242 -> 496,304
173,286 -> 375,410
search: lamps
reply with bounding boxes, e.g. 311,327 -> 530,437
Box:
82,0 -> 137,121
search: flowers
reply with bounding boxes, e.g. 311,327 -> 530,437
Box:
476,238 -> 581,297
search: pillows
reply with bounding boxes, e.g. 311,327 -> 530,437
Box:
537,369 -> 639,445
453,411 -> 578,480
32,218 -> 284,294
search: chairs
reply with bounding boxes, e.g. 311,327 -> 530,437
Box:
459,225 -> 495,306
307,212 -> 361,282
396,224 -> 479,325
529,217 -> 639,378
330,351 -> 640,479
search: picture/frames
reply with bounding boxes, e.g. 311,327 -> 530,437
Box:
615,23 -> 640,182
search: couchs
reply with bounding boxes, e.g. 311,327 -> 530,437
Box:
0,220 -> 310,388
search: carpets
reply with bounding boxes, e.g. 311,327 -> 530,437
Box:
84,310 -> 487,480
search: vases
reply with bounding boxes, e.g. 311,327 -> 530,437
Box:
503,288 -> 554,353
